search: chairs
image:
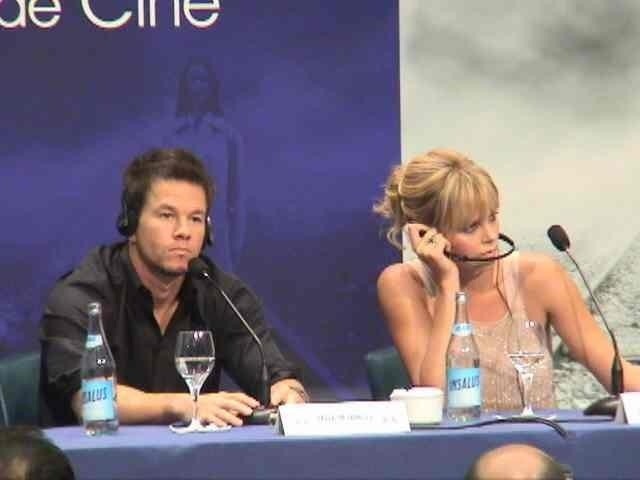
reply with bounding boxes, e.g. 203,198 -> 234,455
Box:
0,349 -> 42,428
364,345 -> 412,403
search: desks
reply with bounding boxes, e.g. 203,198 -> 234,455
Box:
39,407 -> 639,480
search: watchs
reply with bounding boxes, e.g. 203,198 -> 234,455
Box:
284,380 -> 311,406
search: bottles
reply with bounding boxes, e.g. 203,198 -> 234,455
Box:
443,291 -> 483,424
80,300 -> 119,439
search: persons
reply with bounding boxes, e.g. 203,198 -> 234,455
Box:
465,441 -> 574,480
377,150 -> 640,411
37,146 -> 311,429
0,426 -> 75,480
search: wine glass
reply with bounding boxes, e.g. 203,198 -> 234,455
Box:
174,331 -> 216,432
506,320 -> 546,418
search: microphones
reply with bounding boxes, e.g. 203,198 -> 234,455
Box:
187,257 -> 278,426
547,224 -> 626,416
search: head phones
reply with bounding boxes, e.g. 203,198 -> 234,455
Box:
416,229 -> 517,263
115,149 -> 217,248
